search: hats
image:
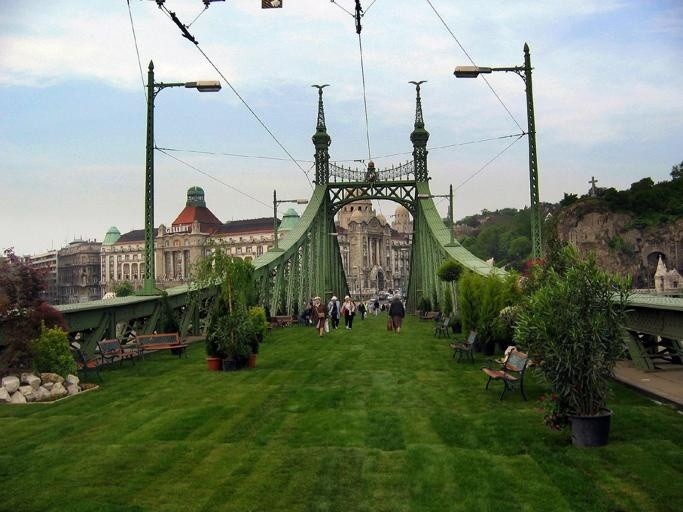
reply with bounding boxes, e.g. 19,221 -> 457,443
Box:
331,295 -> 338,300
313,297 -> 321,301
345,296 -> 349,299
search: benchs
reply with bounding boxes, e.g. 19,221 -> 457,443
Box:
136,332 -> 189,361
449,331 -> 479,364
435,317 -> 450,339
95,339 -> 136,369
478,348 -> 531,402
267,316 -> 300,336
423,311 -> 440,322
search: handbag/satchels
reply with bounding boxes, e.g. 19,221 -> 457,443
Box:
387,319 -> 393,330
351,311 -> 355,317
318,313 -> 324,317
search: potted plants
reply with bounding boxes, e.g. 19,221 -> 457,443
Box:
435,260 -> 464,334
459,271 -> 520,356
185,236 -> 267,370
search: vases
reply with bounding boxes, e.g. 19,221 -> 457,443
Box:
565,409 -> 612,449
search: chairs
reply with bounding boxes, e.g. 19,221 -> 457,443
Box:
68,344 -> 103,384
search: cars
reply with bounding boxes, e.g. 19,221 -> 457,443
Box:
370,293 -> 393,301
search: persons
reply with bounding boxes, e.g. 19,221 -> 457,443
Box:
164,317 -> 182,354
300,294 -> 368,338
366,298 -> 392,317
387,293 -> 405,334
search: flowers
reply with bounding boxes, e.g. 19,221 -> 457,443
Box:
512,243 -> 632,430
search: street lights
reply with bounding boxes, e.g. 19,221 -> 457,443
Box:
136,60 -> 221,295
267,189 -> 309,253
453,41 -> 542,261
417,184 -> 459,248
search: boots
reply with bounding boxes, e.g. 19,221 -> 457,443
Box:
320,327 -> 324,336
